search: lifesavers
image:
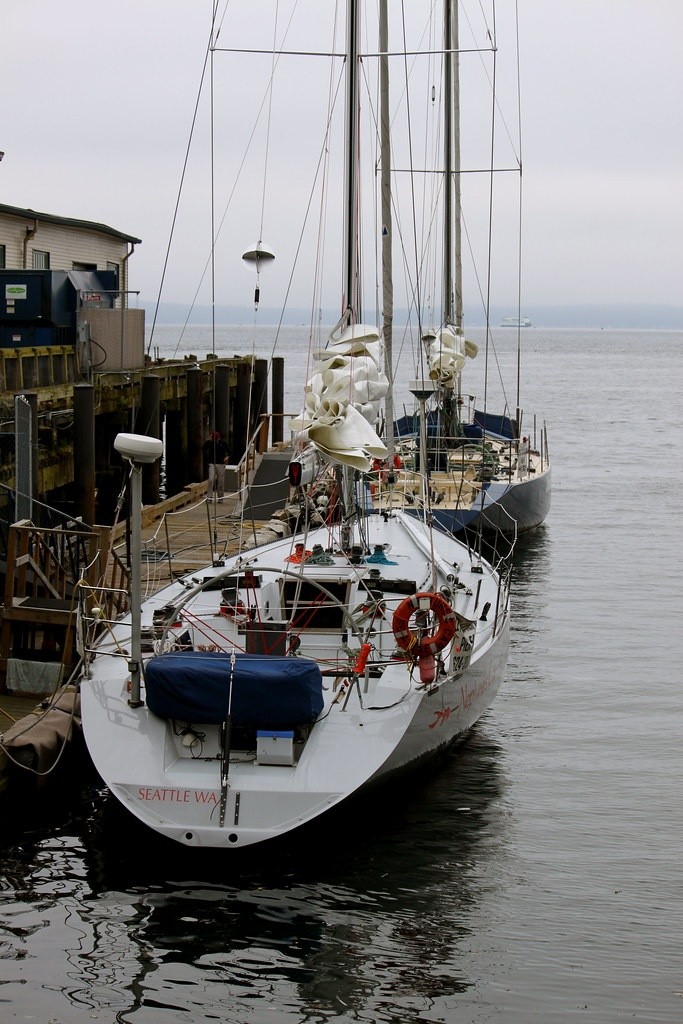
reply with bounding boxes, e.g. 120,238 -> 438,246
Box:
392,592 -> 456,657
373,452 -> 401,481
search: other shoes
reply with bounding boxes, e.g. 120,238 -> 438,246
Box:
218,500 -> 223,503
206,500 -> 212,504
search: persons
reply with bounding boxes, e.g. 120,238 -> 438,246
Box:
203,431 -> 230,504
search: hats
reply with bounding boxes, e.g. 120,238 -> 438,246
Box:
211,432 -> 219,438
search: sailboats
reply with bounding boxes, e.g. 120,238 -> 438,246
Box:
74,0 -> 557,850
